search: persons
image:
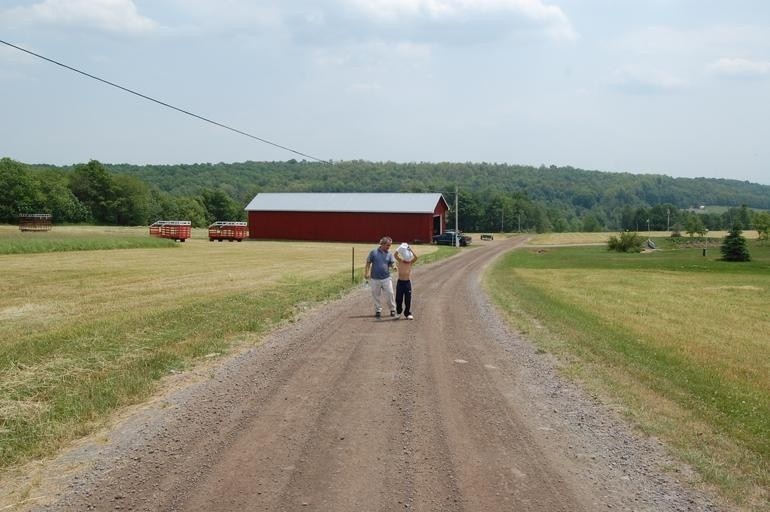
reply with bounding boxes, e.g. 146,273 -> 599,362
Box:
362,236 -> 397,319
393,242 -> 418,320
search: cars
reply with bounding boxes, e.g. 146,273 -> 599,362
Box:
432,231 -> 472,246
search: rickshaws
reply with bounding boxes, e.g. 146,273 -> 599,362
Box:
479,231 -> 495,241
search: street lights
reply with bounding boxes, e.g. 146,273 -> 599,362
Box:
666,207 -> 671,231
705,228 -> 709,255
646,218 -> 650,240
